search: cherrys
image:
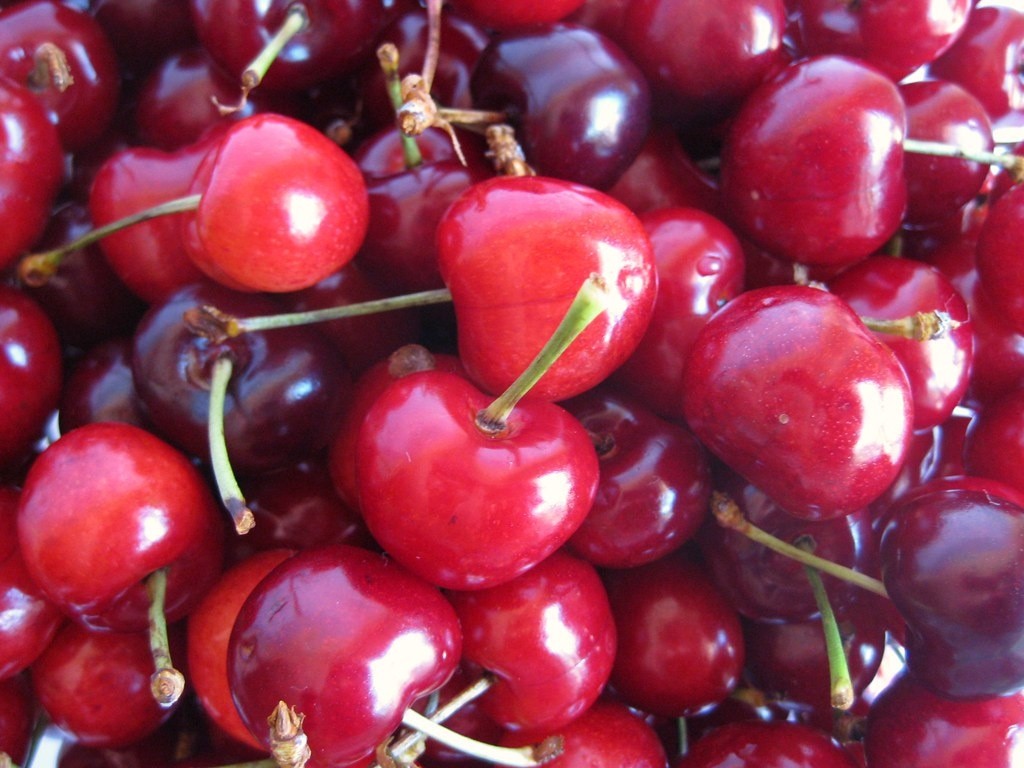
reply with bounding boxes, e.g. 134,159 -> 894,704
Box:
0,0 -> 1024,768
